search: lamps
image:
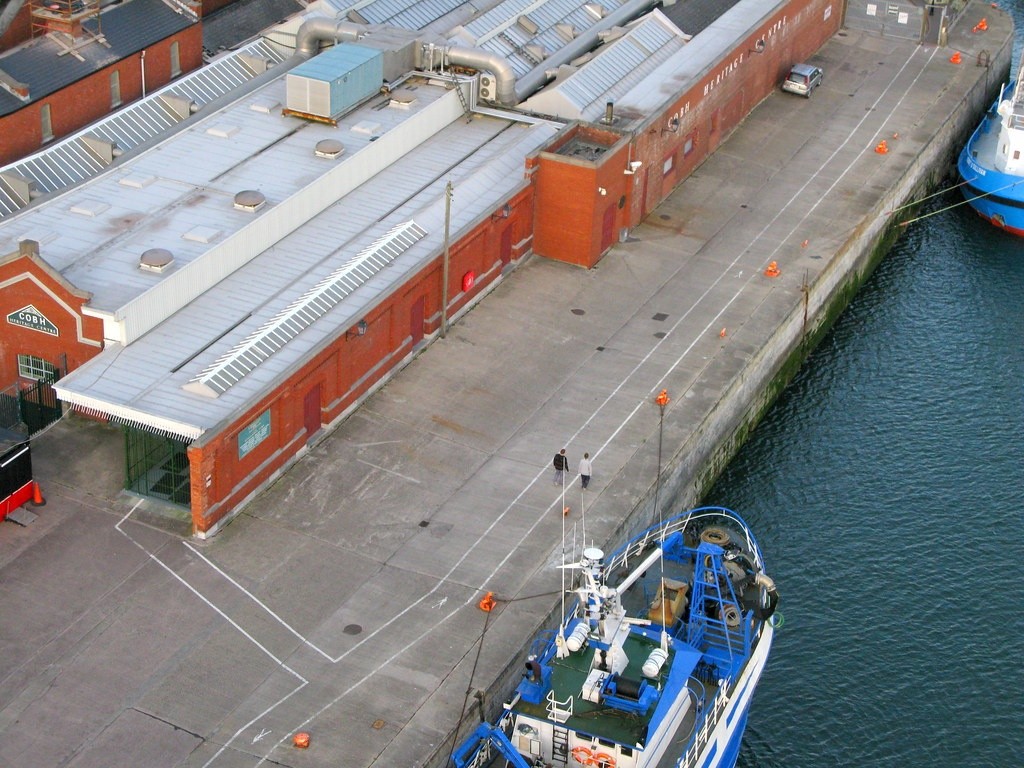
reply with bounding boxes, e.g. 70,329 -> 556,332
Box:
491,203 -> 511,223
748,40 -> 766,58
346,318 -> 368,341
660,118 -> 680,137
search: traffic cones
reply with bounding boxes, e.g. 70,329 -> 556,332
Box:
31,482 -> 46,506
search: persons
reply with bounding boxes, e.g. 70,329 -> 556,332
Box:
552,449 -> 569,486
580,452 -> 592,490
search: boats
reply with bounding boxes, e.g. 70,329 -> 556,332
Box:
956,48 -> 1024,237
451,456 -> 780,768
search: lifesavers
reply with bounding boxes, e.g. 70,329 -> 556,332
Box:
594,754 -> 616,768
572,746 -> 593,765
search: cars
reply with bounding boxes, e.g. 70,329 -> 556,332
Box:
782,64 -> 824,99
